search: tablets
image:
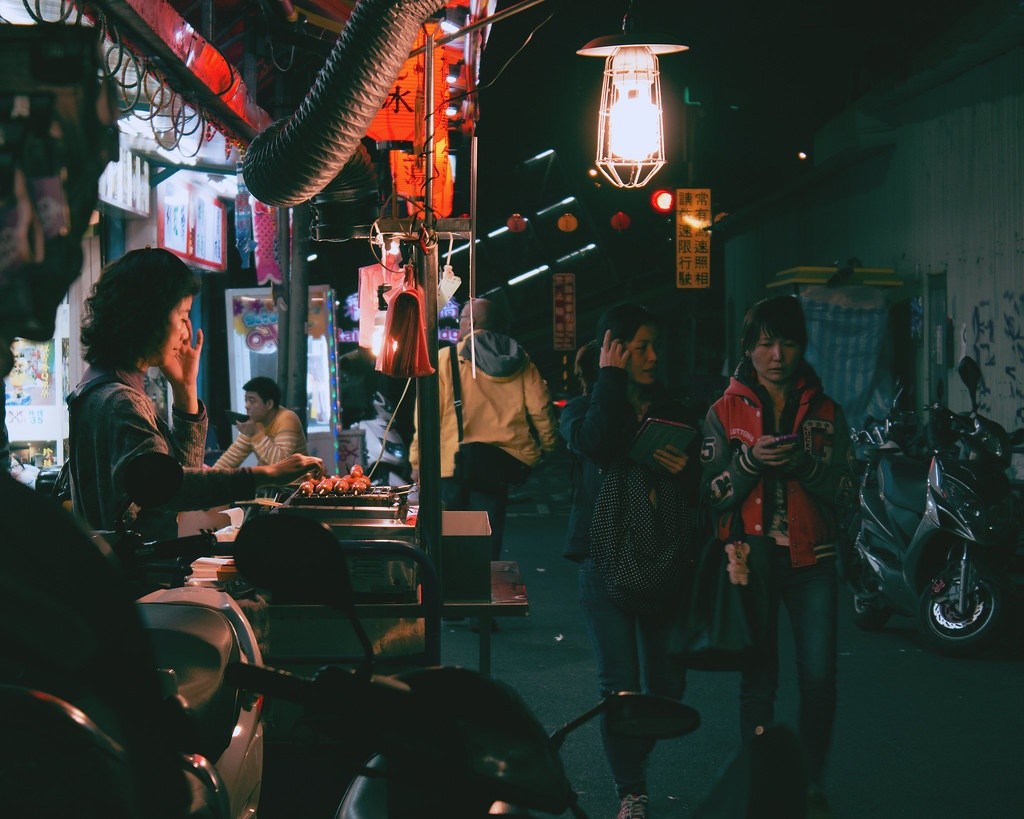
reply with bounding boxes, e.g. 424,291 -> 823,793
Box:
625,417 -> 695,475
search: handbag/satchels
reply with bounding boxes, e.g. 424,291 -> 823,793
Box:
448,443 -> 531,495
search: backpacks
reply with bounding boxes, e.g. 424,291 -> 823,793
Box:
589,402 -> 698,622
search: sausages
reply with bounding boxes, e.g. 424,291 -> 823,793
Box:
297,464 -> 372,499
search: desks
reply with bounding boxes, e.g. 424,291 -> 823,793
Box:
235,558 -> 532,675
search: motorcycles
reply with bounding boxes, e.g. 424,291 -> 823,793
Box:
842,355 -> 1023,658
0,511 -> 702,818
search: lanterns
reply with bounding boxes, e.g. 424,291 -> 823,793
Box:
370,9 -> 457,226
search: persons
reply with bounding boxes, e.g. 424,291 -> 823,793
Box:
557,301 -> 708,818
208,376 -> 310,470
703,293 -> 854,818
407,298 -> 565,635
65,245 -> 327,588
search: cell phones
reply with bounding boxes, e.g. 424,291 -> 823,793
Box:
773,434 -> 799,446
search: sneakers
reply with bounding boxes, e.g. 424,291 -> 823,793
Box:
617,794 -> 648,819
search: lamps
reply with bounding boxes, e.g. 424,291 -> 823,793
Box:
574,1 -> 690,190
